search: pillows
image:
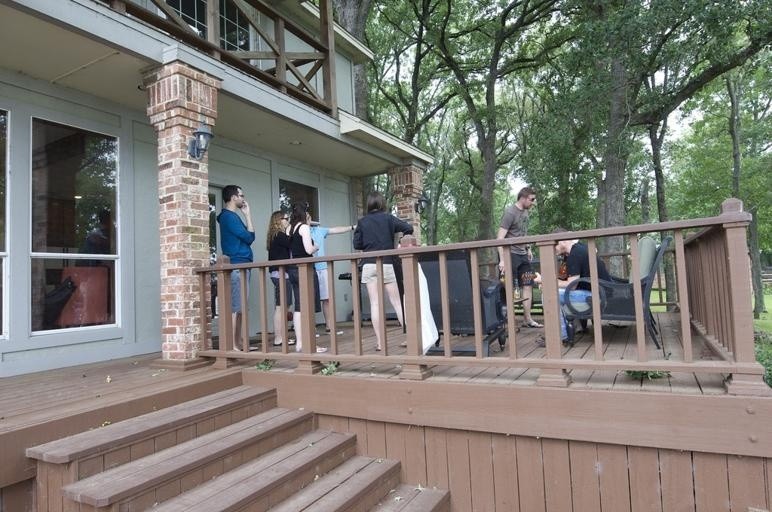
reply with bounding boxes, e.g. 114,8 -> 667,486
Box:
629,237 -> 656,285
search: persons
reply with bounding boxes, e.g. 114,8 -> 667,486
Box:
76,210 -> 111,267
265,210 -> 296,346
532,226 -> 616,348
352,189 -> 416,350
214,184 -> 261,352
291,212 -> 358,339
491,188 -> 544,333
287,198 -> 329,354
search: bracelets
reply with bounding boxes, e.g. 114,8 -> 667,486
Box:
352,225 -> 354,230
526,246 -> 530,250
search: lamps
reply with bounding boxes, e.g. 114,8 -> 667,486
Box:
188,121 -> 215,160
415,193 -> 428,214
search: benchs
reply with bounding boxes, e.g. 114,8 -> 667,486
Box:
565,236 -> 673,349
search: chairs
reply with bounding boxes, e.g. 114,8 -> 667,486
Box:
392,248 -> 508,359
51,266 -> 109,328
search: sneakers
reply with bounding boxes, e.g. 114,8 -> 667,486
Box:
315,329 -> 344,353
535,337 -> 569,347
521,319 -> 544,328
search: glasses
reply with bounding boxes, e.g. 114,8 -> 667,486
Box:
281,217 -> 289,221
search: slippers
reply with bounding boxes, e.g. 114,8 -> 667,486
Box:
273,338 -> 296,346
